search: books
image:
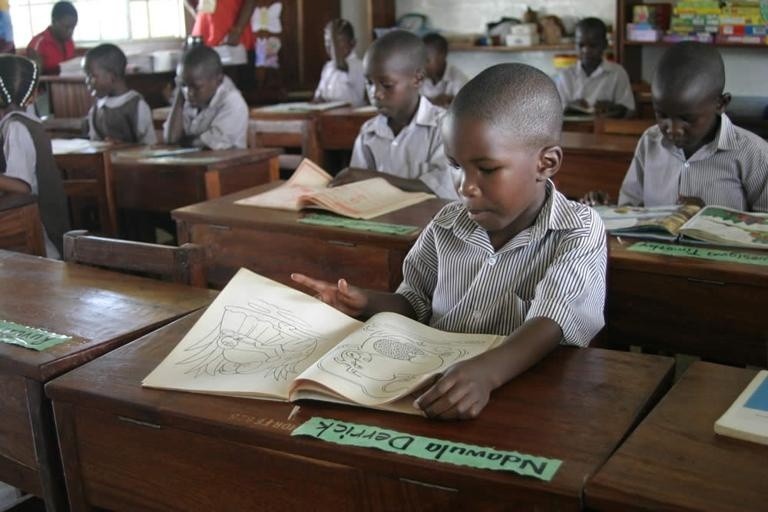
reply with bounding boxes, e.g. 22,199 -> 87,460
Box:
591,203 -> 768,251
140,266 -> 506,418
260,101 -> 351,113
664,0 -> 768,45
713,369 -> 768,445
562,103 -> 619,121
234,157 -> 436,222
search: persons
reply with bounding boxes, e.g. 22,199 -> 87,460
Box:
0,52 -> 73,259
162,45 -> 248,151
326,29 -> 465,199
26,1 -> 78,73
421,33 -> 470,110
617,39 -> 768,217
309,18 -> 367,108
289,62 -> 607,426
556,18 -> 637,121
80,42 -> 159,146
182,0 -> 257,106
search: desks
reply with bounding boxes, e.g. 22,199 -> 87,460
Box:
0,63 -> 768,512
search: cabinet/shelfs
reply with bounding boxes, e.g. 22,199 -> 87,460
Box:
619,0 -> 768,83
